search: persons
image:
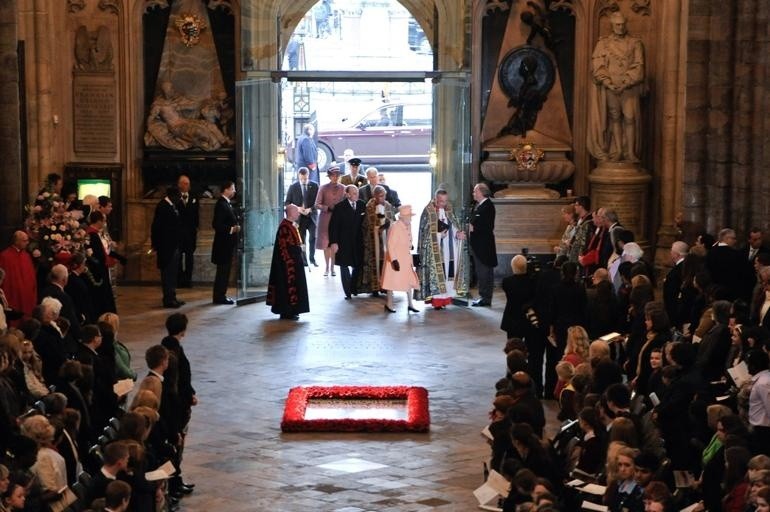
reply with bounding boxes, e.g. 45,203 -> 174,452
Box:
264,203 -> 311,321
340,158 -> 367,187
469,183 -> 498,306
489,195 -> 769,511
286,169 -> 318,268
366,108 -> 390,127
1,174 -> 196,512
211,179 -> 241,304
413,190 -> 467,310
380,205 -> 421,313
377,172 -> 386,185
147,82 -> 234,151
362,186 -> 394,297
327,184 -> 365,301
314,166 -> 346,276
359,167 -> 401,207
591,11 -> 646,163
298,125 -> 322,184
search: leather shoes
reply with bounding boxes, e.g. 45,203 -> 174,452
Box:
473,297 -> 491,306
174,492 -> 184,498
181,486 -> 193,493
212,297 -> 235,304
453,298 -> 468,305
310,262 -> 318,267
164,302 -> 179,307
280,315 -> 299,320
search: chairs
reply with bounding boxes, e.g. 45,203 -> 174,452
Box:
21,383 -> 61,416
633,393 -> 675,482
71,401 -> 129,506
553,437 -> 579,465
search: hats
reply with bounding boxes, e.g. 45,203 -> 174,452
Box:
746,455 -> 769,468
348,158 -> 361,165
326,167 -> 344,175
398,205 -> 416,216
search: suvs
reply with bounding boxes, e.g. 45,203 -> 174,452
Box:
285,101 -> 432,173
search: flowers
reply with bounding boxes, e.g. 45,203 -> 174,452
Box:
23,190 -> 93,277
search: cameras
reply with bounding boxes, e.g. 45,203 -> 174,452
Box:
523,302 -> 541,329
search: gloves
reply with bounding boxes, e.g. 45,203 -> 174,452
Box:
328,207 -> 332,212
392,260 -> 399,271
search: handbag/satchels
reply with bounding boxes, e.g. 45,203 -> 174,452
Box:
438,220 -> 450,232
412,254 -> 422,266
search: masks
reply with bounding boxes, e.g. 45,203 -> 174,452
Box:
748,470 -> 767,481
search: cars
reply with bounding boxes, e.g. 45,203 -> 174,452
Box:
408,14 -> 435,56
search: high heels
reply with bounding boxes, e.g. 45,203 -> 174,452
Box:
323,271 -> 329,276
331,271 -> 336,276
384,303 -> 396,313
408,306 -> 419,312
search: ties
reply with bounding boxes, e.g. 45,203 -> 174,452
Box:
303,184 -> 307,209
182,195 -> 187,207
352,203 -> 355,209
